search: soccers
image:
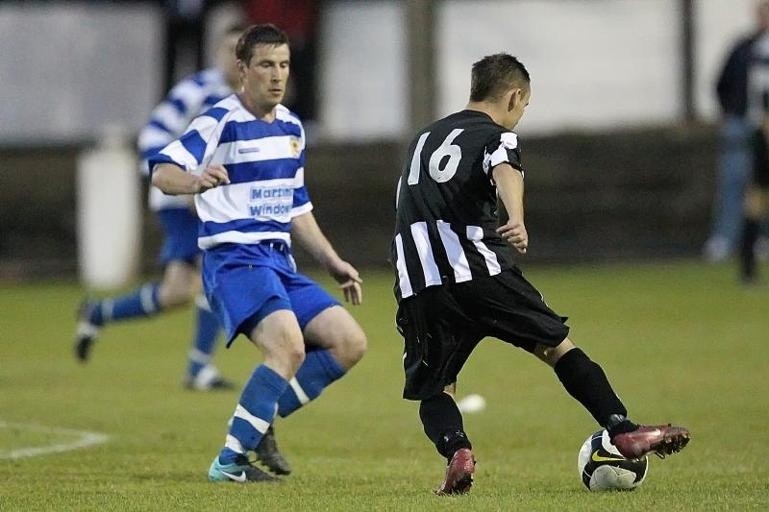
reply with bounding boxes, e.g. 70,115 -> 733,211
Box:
579,430 -> 649,491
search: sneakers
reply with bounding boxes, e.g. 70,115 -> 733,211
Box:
74,299 -> 97,360
611,423 -> 692,459
208,455 -> 276,481
183,364 -> 234,392
255,419 -> 291,474
435,449 -> 475,494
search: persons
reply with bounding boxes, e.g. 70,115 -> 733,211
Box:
388,53 -> 692,494
145,22 -> 369,489
70,12 -> 257,391
696,0 -> 769,269
737,88 -> 768,279
